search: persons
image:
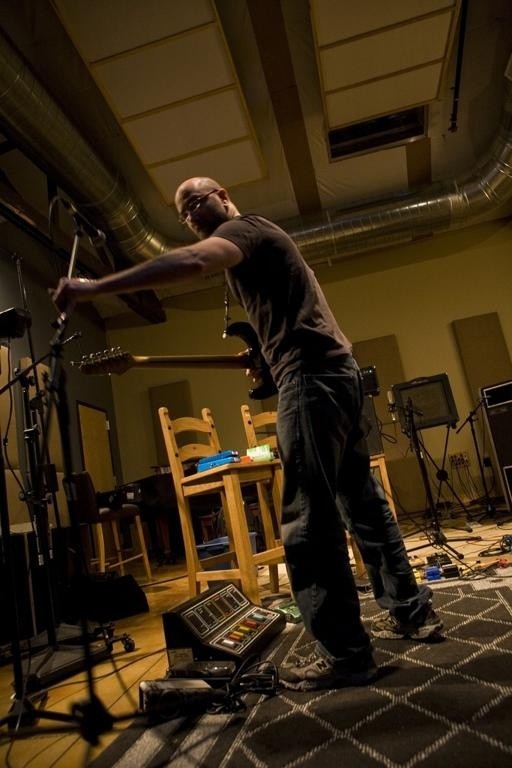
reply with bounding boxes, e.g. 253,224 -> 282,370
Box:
48,176 -> 445,690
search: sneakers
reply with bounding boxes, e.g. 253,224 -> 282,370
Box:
370,610 -> 444,640
277,642 -> 379,692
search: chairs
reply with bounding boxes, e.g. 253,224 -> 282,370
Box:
241,405 -> 398,593
159,405 -> 287,609
63,472 -> 153,581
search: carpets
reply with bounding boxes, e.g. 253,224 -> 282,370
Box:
77,561 -> 512,767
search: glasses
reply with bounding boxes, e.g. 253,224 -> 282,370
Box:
177,189 -> 219,225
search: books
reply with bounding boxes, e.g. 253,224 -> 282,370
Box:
193,450 -> 240,471
